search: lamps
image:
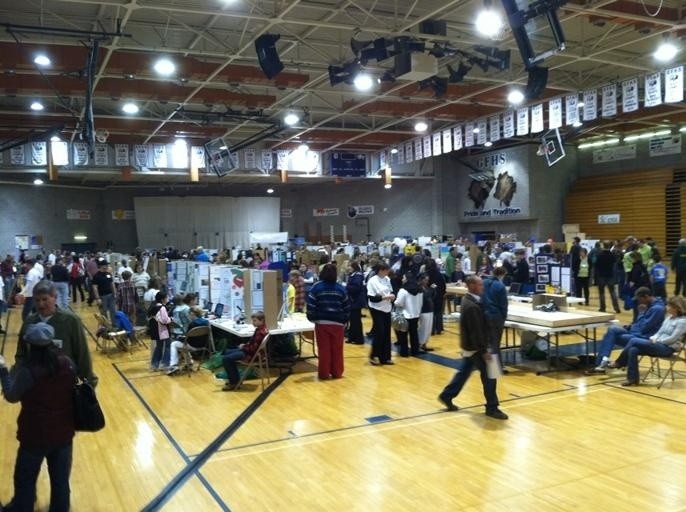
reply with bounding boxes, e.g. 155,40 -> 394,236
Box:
336,177 -> 341,184
122,167 -> 131,180
281,170 -> 287,183
48,143 -> 57,180
191,166 -> 199,181
385,168 -> 392,188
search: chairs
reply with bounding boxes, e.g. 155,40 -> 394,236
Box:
115,311 -> 148,351
102,316 -> 132,355
94,313 -> 120,351
622,356 -> 654,372
642,334 -> 686,389
176,326 -> 210,378
233,333 -> 271,391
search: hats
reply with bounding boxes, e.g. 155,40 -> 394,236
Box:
97,257 -> 110,266
24,323 -> 55,346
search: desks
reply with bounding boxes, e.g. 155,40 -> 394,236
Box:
202,312 -> 319,358
445,286 -> 469,314
499,305 -> 619,375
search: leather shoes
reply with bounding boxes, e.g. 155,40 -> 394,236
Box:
351,340 -> 362,344
419,347 -> 427,353
424,346 -> 435,352
344,338 -> 353,343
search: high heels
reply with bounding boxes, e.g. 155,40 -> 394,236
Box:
621,379 -> 640,387
608,362 -> 623,369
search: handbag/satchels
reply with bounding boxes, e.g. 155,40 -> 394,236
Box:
390,302 -> 409,332
67,359 -> 105,432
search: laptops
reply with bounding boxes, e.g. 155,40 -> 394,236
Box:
208,303 -> 224,318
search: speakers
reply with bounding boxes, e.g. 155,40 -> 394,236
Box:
523,65 -> 549,100
255,33 -> 285,80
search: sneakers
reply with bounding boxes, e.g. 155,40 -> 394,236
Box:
382,359 -> 394,365
222,383 -> 241,391
484,404 -> 509,420
437,394 -> 458,411
584,367 -> 607,375
166,365 -> 179,375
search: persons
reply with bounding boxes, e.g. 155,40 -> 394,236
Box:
585,286 -> 668,376
537,236 -> 570,267
345,262 -> 368,346
439,275 -> 510,420
137,241 -> 269,273
514,237 -> 530,285
570,234 -> 669,312
608,296 -> 686,387
299,244 -> 306,256
325,236 -> 354,265
366,263 -> 396,366
20,259 -> 39,320
32,254 -> 46,282
285,268 -> 307,313
430,235 -> 471,307
221,311 -> 270,392
306,264 -> 352,380
170,295 -> 186,335
166,307 -> 209,376
394,272 -> 424,357
0,252 -> 16,309
417,273 -> 438,354
38,245 -> 47,262
1,322 -> 80,511
68,248 -> 97,306
180,293 -> 197,326
0,275 -> 9,338
672,240 -> 686,301
281,273 -> 297,318
15,281 -> 95,391
93,254 -> 149,348
148,291 -> 170,371
51,256 -> 69,309
480,267 -> 508,374
475,239 -> 519,289
354,234 -> 431,274
527,256 -> 535,283
